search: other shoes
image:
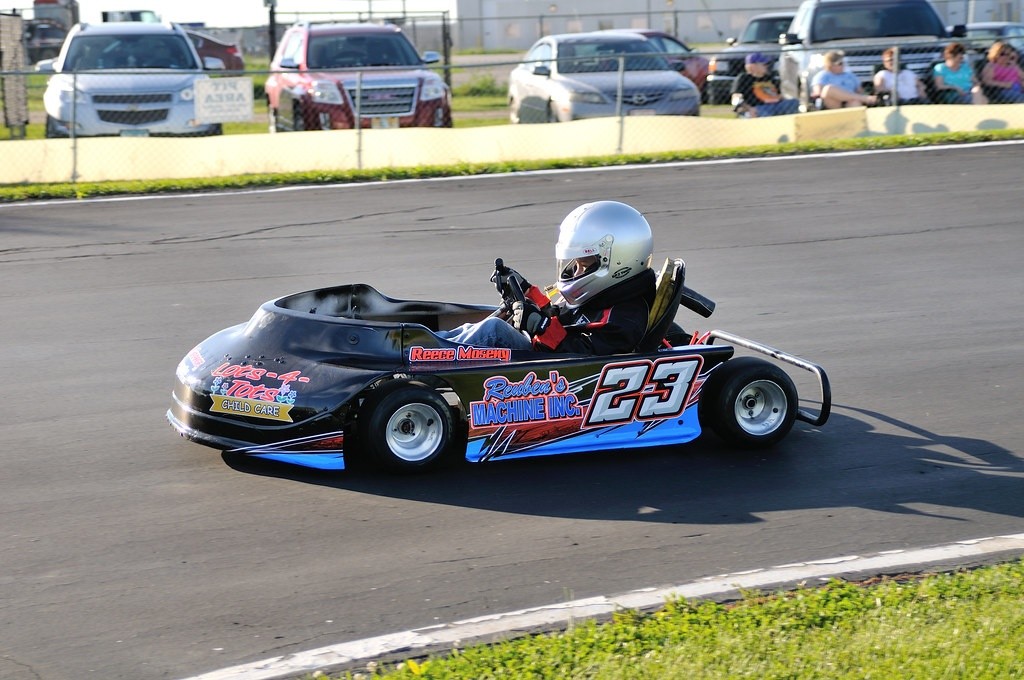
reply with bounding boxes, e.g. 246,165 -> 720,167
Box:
866,91 -> 891,107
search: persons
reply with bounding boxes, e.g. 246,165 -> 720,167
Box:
730,53 -> 799,119
811,50 -> 889,110
873,49 -> 931,106
407,201 -> 657,359
981,42 -> 1024,103
933,42 -> 989,105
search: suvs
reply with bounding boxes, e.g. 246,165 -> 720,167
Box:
706,11 -> 836,105
264,19 -> 453,133
778,0 -> 967,114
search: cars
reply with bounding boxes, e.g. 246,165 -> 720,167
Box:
508,30 -> 700,124
35,29 -> 246,78
945,22 -> 1023,66
43,22 -> 223,138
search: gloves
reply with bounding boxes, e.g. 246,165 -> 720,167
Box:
512,296 -> 551,336
489,266 -> 531,301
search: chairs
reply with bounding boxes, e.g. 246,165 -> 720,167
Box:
310,44 -> 327,59
152,47 -> 170,60
564,257 -> 686,352
75,44 -> 108,69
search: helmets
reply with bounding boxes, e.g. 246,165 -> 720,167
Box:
555,199 -> 653,303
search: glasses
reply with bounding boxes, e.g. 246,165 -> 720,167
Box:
1000,51 -> 1011,56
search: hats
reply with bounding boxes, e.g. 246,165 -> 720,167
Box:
745,52 -> 769,63
824,48 -> 845,66
